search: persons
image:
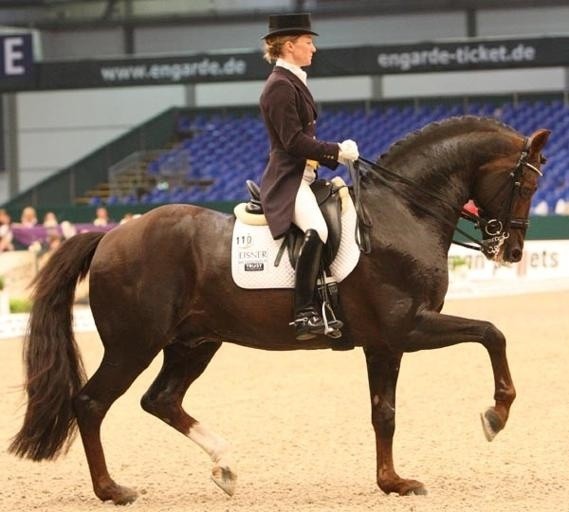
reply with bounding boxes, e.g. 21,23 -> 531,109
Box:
0,205 -> 113,256
259,12 -> 359,341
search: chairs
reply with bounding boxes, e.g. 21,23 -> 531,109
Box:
88,99 -> 569,215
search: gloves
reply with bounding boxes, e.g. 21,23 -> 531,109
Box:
338,138 -> 360,167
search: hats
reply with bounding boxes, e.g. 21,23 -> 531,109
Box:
260,13 -> 319,39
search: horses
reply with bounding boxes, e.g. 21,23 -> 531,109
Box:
8,115 -> 551,505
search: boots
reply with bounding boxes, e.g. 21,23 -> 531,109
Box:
292,229 -> 342,340
